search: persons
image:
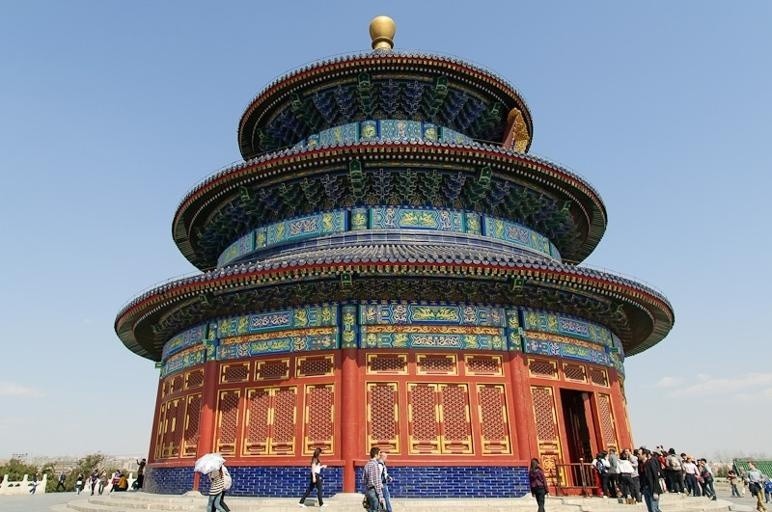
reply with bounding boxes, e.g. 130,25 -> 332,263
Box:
727,461 -> 772,512
207,466 -> 226,512
30,472 -> 39,496
361,447 -> 387,512
298,448 -> 328,508
528,459 -> 548,512
591,445 -> 717,512
212,465 -> 232,512
75,459 -> 146,494
55,470 -> 66,492
377,452 -> 392,512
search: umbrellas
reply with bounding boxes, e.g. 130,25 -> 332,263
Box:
193,451 -> 227,474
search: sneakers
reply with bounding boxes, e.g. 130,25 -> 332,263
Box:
298,502 -> 306,508
